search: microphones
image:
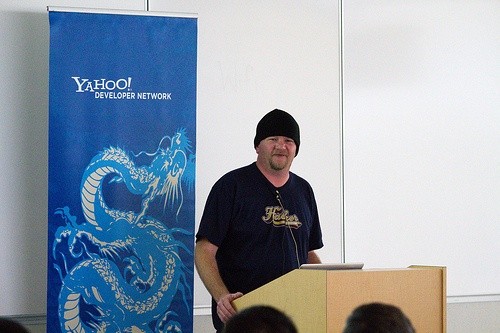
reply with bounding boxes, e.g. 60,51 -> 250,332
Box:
271,188 -> 300,268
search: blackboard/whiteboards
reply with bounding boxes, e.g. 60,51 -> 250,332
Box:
0,0 -> 500,321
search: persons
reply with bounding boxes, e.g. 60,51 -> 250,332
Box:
343,303 -> 415,333
223,305 -> 298,333
0,318 -> 28,333
195,109 -> 324,333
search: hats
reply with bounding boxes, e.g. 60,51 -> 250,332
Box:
254,108 -> 299,157
